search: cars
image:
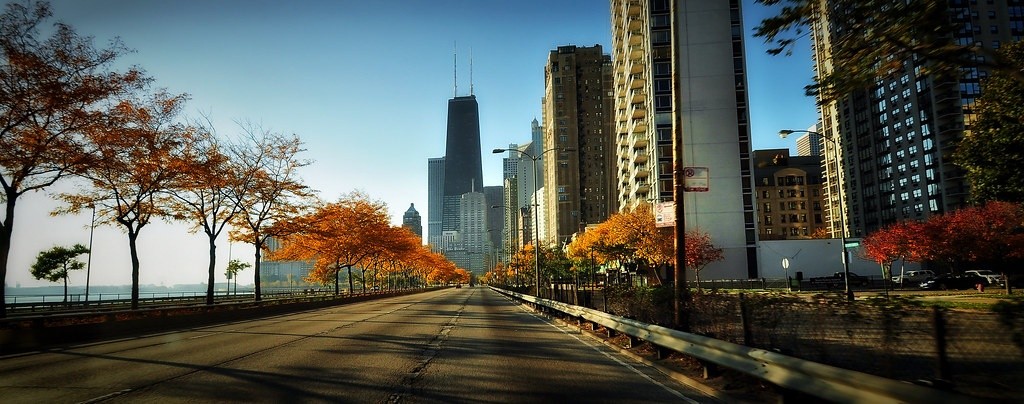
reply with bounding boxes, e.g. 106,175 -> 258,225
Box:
889,260 -> 1009,293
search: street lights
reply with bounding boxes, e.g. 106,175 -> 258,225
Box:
779,127 -> 856,303
490,201 -> 541,288
493,143 -> 577,297
486,226 -> 527,286
